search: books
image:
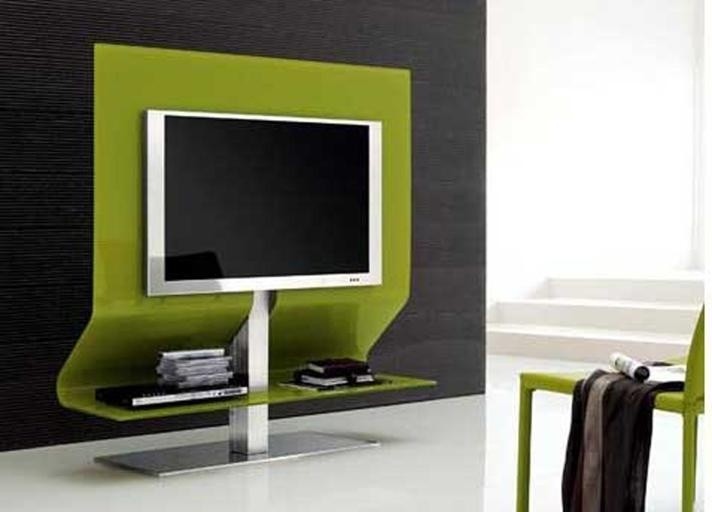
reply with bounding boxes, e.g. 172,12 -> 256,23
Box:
278,356 -> 374,392
95,344 -> 248,408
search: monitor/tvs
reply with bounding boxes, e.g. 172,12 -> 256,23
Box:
145,109 -> 383,297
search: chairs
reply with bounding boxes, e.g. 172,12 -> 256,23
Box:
517,304 -> 704,511
